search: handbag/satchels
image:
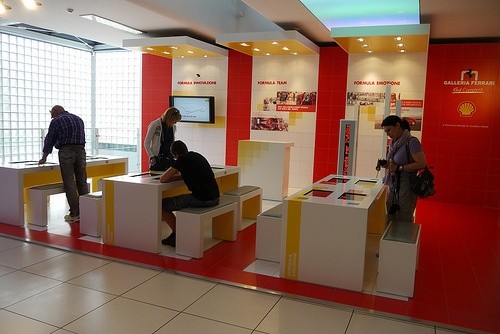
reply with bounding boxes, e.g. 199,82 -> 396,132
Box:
410,166 -> 435,198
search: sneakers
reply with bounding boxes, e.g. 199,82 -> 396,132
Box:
64,214 -> 80,221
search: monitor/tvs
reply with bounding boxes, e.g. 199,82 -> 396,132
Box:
169,96 -> 215,124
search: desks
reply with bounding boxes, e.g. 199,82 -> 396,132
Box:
281,173 -> 389,291
99,164 -> 242,255
0,155 -> 129,227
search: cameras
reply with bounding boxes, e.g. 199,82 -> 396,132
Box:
376,159 -> 388,171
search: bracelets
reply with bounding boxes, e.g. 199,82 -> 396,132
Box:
396,165 -> 404,173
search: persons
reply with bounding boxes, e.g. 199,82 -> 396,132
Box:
159,140 -> 221,248
143,106 -> 182,170
380,114 -> 428,224
37,105 -> 89,222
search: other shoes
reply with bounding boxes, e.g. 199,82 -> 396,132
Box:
162,232 -> 176,245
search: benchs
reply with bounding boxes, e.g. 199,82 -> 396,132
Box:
223,185 -> 263,230
176,198 -> 239,259
255,202 -> 284,263
376,221 -> 422,299
79,191 -> 102,238
25,181 -> 90,226
